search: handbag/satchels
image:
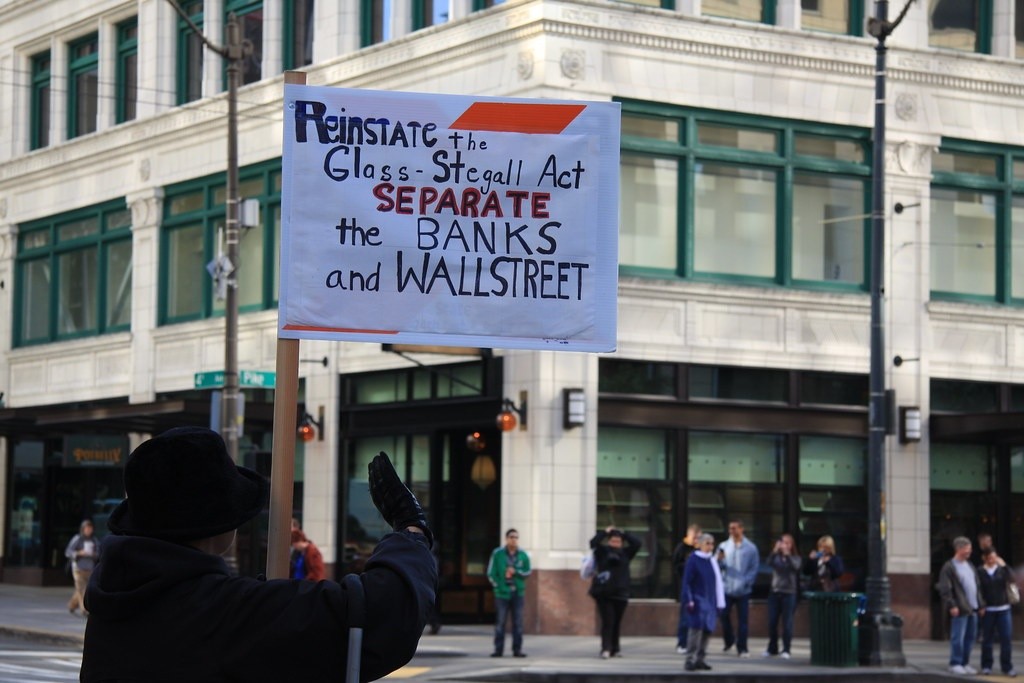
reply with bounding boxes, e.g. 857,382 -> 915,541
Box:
1005,580 -> 1021,604
580,550 -> 596,579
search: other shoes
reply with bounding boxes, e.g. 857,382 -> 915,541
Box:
613,652 -> 624,658
1007,669 -> 1017,677
982,668 -> 991,675
949,664 -> 966,675
513,650 -> 526,657
696,661 -> 710,670
965,665 -> 977,674
739,652 -> 749,658
602,650 -> 609,658
762,651 -> 772,656
685,661 -> 694,670
491,650 -> 501,656
782,652 -> 790,659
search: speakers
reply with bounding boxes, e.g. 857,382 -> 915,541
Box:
242,198 -> 260,227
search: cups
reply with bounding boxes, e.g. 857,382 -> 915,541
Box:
83,541 -> 95,554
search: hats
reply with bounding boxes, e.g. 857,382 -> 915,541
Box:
106,427 -> 271,543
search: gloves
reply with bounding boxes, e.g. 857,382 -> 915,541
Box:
367,451 -> 434,550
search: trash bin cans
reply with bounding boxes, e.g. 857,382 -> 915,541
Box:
809,592 -> 864,667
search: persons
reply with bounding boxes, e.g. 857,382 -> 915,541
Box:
673,524 -> 702,653
488,529 -> 530,655
977,546 -> 1018,677
805,535 -> 853,591
678,534 -> 726,670
940,533 -> 992,674
718,518 -> 759,657
65,521 -> 99,615
80,426 -> 438,683
762,534 -> 800,657
290,517 -> 322,580
590,526 -> 641,658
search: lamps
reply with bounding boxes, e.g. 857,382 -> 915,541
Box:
898,405 -> 922,446
560,387 -> 585,431
298,405 -> 325,442
495,390 -> 527,432
